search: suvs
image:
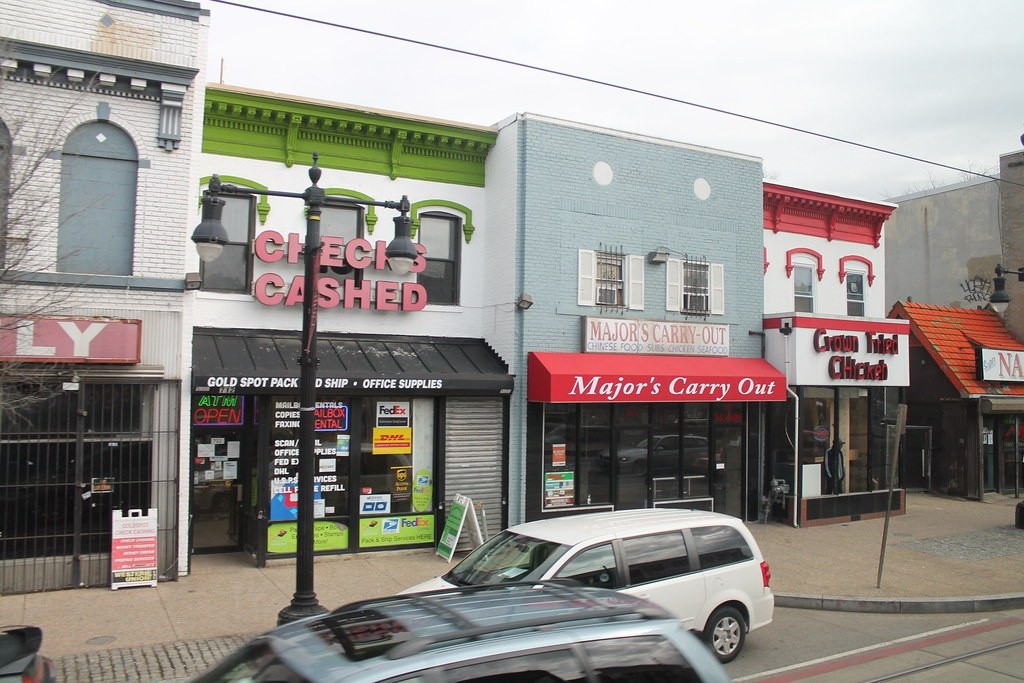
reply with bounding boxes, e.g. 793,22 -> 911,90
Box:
595,434 -> 711,473
0,442 -> 149,518
186,576 -> 734,683
390,506 -> 774,665
241,441 -> 412,514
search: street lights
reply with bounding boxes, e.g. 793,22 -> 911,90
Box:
191,150 -> 419,620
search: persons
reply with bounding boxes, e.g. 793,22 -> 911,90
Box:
825,439 -> 846,495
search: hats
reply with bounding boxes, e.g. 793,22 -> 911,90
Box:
833,439 -> 845,445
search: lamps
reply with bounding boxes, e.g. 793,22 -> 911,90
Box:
518,294 -> 532,309
186,273 -> 201,290
